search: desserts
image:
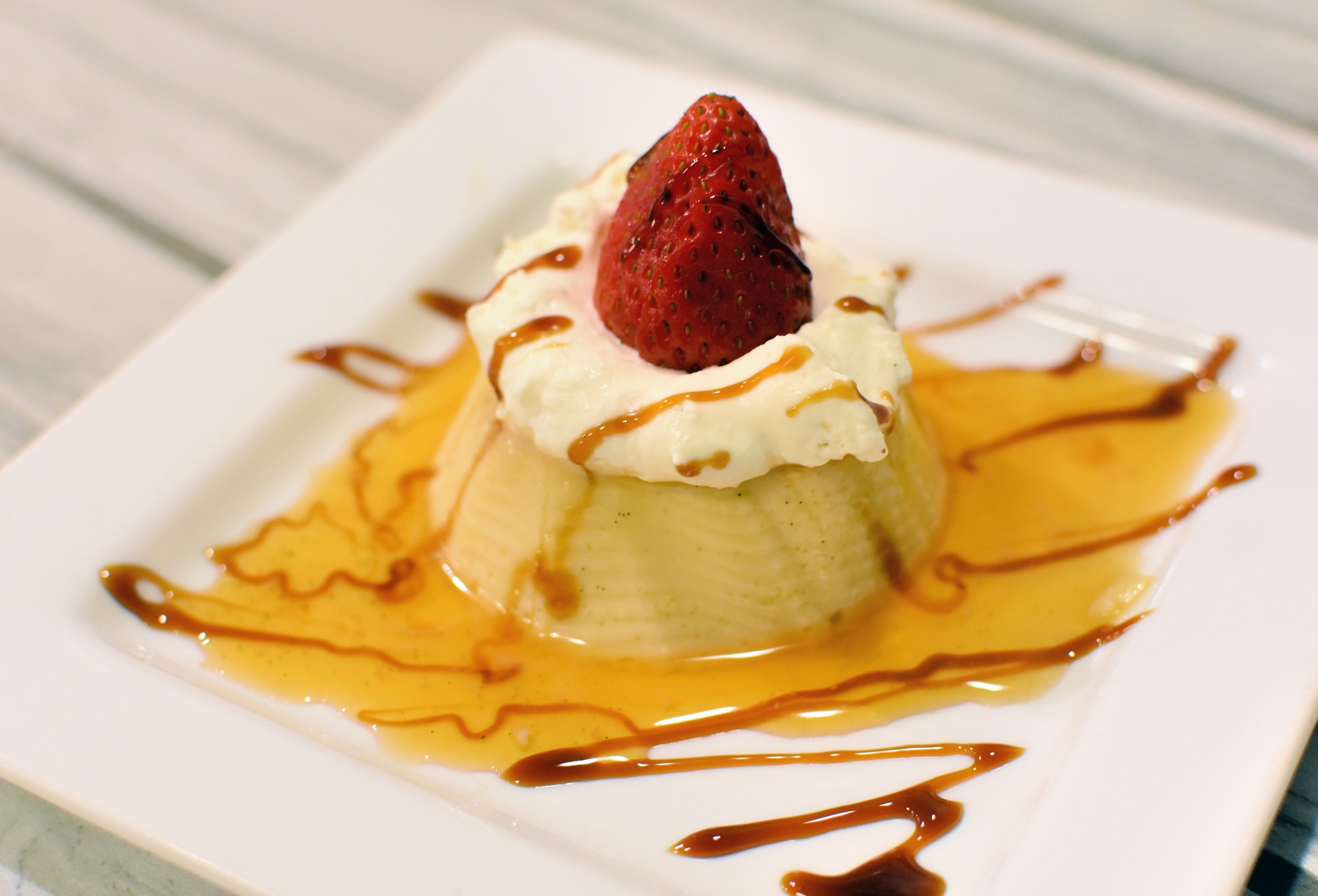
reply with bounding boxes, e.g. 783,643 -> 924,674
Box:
426,92 -> 948,659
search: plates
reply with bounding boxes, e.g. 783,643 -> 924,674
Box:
0,27 -> 1318,896
